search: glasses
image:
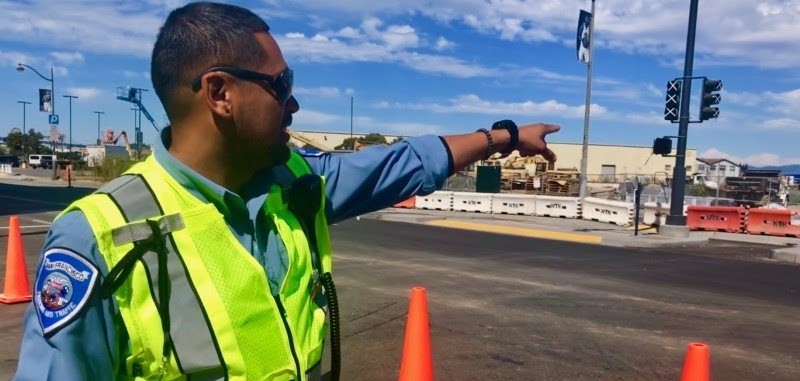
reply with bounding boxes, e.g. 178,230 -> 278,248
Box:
191,65 -> 295,109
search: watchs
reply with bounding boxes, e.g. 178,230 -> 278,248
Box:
492,120 -> 518,154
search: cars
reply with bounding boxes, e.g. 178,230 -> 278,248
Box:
501,155 -> 545,190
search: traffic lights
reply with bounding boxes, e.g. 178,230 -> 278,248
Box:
699,78 -> 722,122
665,80 -> 681,122
653,137 -> 672,155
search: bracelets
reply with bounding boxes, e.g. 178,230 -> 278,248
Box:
475,128 -> 492,160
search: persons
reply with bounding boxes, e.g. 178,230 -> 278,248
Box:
42,91 -> 50,110
11,3 -> 562,381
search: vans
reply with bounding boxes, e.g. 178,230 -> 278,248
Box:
28,154 -> 53,169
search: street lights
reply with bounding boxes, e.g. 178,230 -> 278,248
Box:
63,95 -> 79,164
17,101 -> 32,167
130,107 -> 140,163
16,62 -> 58,181
94,111 -> 104,146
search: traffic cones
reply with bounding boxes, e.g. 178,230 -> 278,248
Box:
0,216 -> 34,304
399,286 -> 434,381
680,342 -> 710,381
